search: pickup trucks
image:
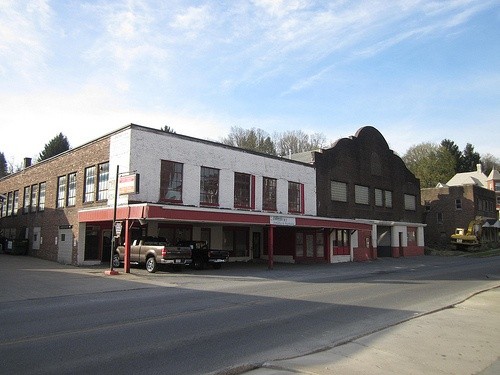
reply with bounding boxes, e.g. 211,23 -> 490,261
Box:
113,236 -> 193,273
177,240 -> 229,269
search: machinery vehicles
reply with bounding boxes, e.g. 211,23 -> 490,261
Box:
450,219 -> 485,252
